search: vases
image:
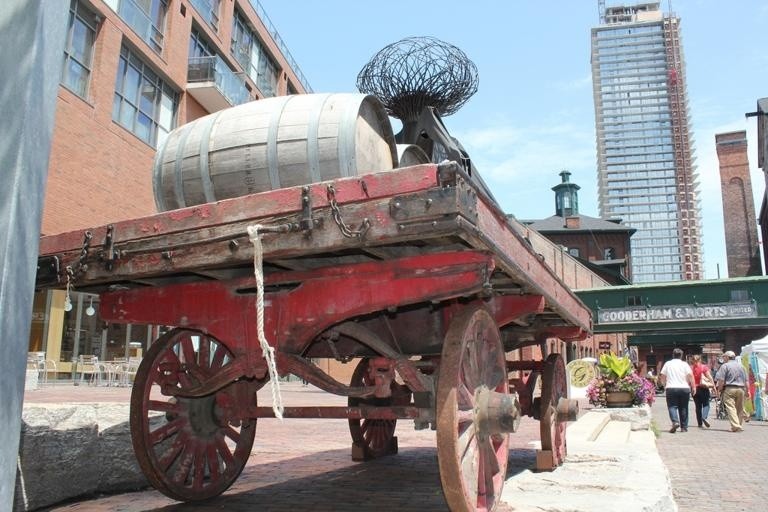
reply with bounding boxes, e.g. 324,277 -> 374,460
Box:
605,389 -> 636,409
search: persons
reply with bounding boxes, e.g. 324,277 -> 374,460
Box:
647,368 -> 653,377
650,367 -> 656,376
716,350 -> 751,433
658,348 -> 697,433
687,353 -> 716,428
705,357 -> 725,403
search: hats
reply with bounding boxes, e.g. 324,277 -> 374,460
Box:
721,350 -> 736,358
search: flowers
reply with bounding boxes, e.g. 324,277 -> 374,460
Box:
584,373 -> 656,407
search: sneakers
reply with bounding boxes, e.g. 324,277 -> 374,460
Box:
681,427 -> 688,432
698,418 -> 710,427
670,423 -> 680,433
728,425 -> 744,432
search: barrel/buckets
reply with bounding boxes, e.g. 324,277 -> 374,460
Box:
606,391 -> 639,407
151,93 -> 399,213
395,140 -> 430,166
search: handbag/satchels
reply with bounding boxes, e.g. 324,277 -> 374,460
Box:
700,372 -> 714,388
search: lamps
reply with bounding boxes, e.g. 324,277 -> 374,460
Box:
85,294 -> 95,317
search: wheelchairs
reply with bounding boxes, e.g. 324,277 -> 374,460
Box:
714,392 -> 726,420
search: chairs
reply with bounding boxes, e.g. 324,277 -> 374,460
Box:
28,349 -> 57,389
79,354 -> 147,387
